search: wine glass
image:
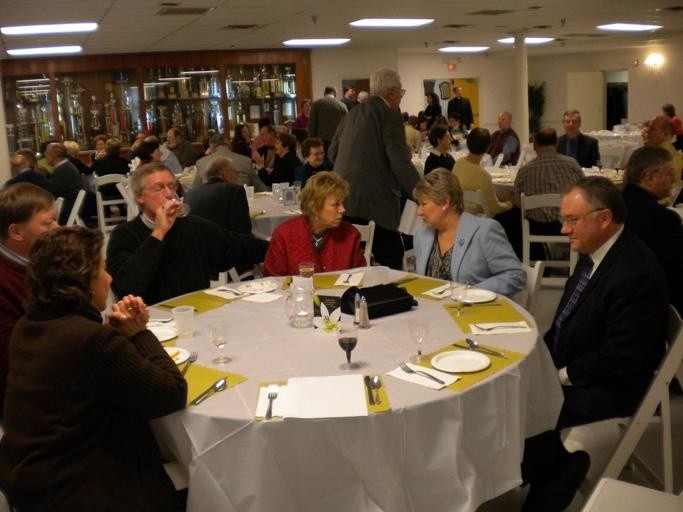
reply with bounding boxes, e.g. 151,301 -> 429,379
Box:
337,324 -> 361,370
162,190 -> 190,217
450,280 -> 468,320
209,323 -> 229,364
411,321 -> 428,361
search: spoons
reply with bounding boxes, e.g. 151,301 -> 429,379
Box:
371,376 -> 382,404
194,379 -> 225,405
465,337 -> 506,359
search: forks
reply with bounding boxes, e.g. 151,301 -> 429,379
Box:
473,323 -> 527,331
180,351 -> 197,377
265,383 -> 277,420
431,285 -> 457,295
399,361 -> 445,384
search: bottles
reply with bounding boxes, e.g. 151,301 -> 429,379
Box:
353,293 -> 370,327
146,67 -> 222,142
68,90 -> 133,151
40,107 -> 51,143
223,64 -> 297,139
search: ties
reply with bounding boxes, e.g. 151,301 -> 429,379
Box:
551,257 -> 593,346
570,140 -> 576,155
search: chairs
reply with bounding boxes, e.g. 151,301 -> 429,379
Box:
53,172 -> 137,239
518,187 -> 580,289
559,301 -> 683,511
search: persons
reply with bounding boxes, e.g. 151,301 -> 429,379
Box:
622,105 -> 682,315
405,109 -> 600,292
515,173 -> 670,510
424,86 -> 475,129
292,70 -> 422,270
1,185 -> 58,374
104,162 -> 222,306
2,225 -> 188,510
3,128 -> 199,224
180,115 -> 367,281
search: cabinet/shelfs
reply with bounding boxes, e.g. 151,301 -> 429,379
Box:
1,48 -> 312,168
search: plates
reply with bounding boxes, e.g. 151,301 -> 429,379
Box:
145,323 -> 179,341
430,351 -> 491,373
457,289 -> 496,302
163,346 -> 189,364
487,166 -> 624,184
238,281 -> 277,293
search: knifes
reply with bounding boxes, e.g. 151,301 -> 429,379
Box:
188,376 -> 227,405
158,304 -> 198,312
453,343 -> 500,356
364,375 -> 375,404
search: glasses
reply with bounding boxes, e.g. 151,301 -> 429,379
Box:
138,182 -> 179,198
393,87 -> 406,98
558,207 -> 606,227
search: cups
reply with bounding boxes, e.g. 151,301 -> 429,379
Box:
171,306 -> 193,338
285,276 -> 316,328
243,181 -> 300,206
590,124 -> 644,148
298,262 -> 313,278
